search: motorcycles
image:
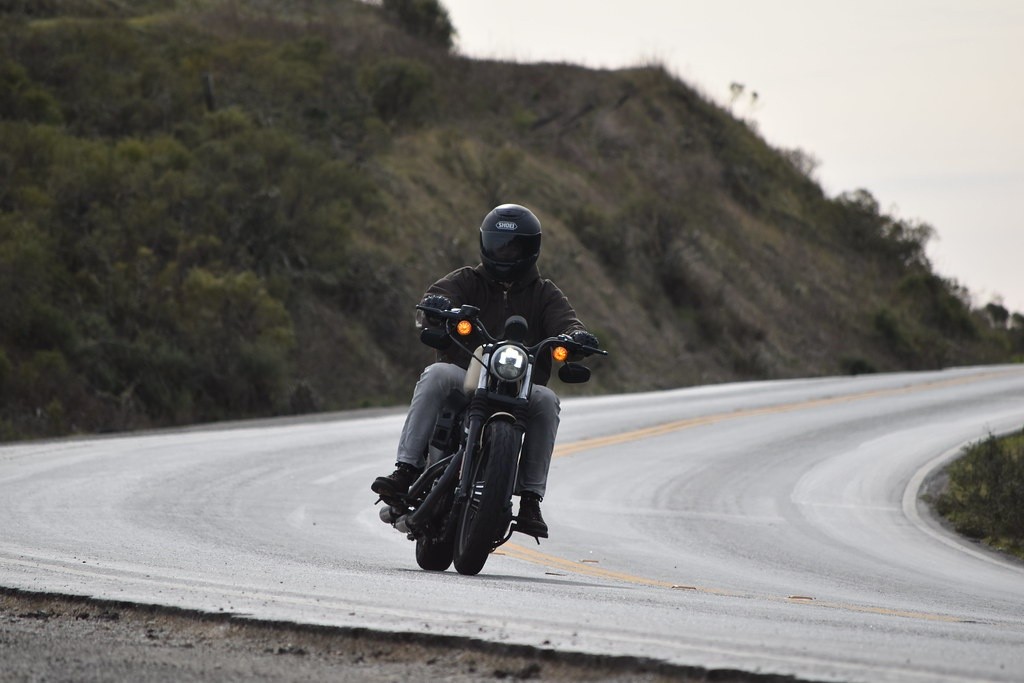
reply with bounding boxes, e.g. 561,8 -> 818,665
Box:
380,304 -> 609,575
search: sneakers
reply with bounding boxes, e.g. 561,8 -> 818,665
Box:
516,500 -> 548,535
370,465 -> 424,497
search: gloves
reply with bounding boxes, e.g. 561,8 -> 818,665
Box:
421,293 -> 452,320
558,329 -> 600,357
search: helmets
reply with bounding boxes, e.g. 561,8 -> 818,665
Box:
479,203 -> 542,280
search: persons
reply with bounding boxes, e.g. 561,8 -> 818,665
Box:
370,204 -> 600,539
